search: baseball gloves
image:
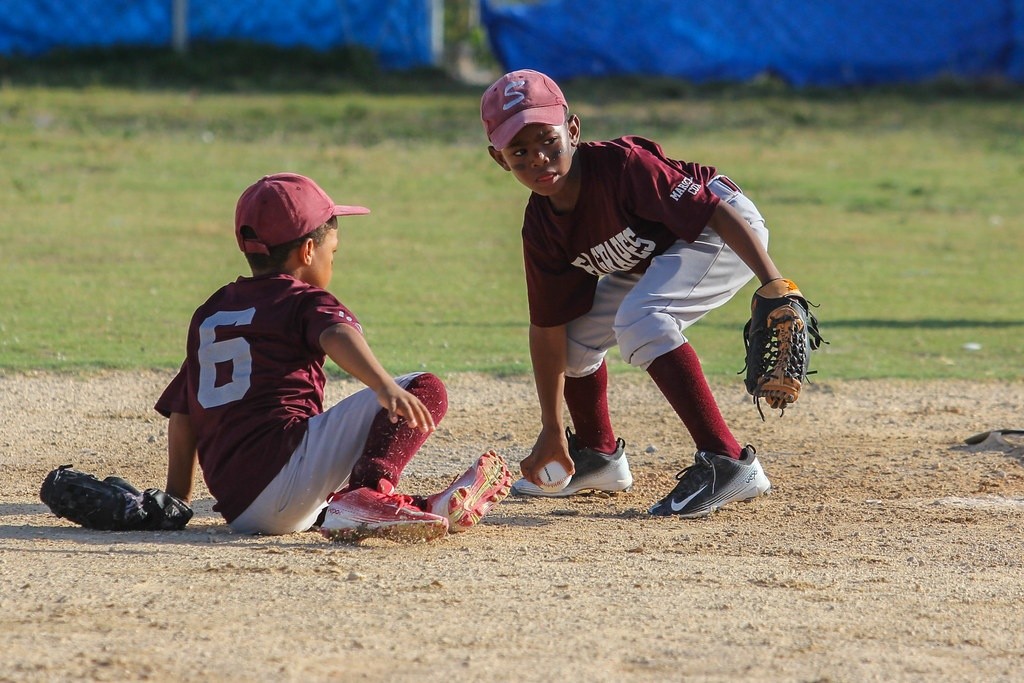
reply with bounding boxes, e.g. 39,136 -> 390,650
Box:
742,278 -> 821,410
39,464 -> 194,531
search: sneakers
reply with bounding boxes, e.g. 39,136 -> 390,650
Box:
424,450 -> 512,533
320,478 -> 449,544
647,443 -> 774,519
510,425 -> 634,497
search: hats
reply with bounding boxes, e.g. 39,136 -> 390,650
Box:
235,172 -> 371,257
480,69 -> 569,151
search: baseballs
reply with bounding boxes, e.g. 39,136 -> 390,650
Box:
534,460 -> 573,493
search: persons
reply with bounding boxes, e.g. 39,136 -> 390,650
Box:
154,172 -> 513,547
481,68 -> 830,520
